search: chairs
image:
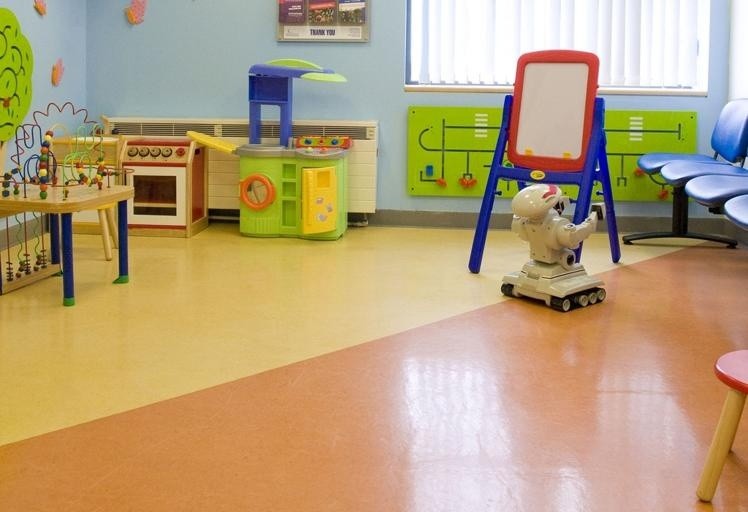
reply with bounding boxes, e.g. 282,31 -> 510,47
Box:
621,92 -> 747,253
41,131 -> 130,259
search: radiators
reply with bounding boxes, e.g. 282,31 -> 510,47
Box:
102,115 -> 381,230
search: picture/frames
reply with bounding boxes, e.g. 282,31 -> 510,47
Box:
276,0 -> 371,45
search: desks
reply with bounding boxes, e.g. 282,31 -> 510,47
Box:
0,182 -> 135,307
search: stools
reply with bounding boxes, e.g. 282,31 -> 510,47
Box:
695,349 -> 747,503
